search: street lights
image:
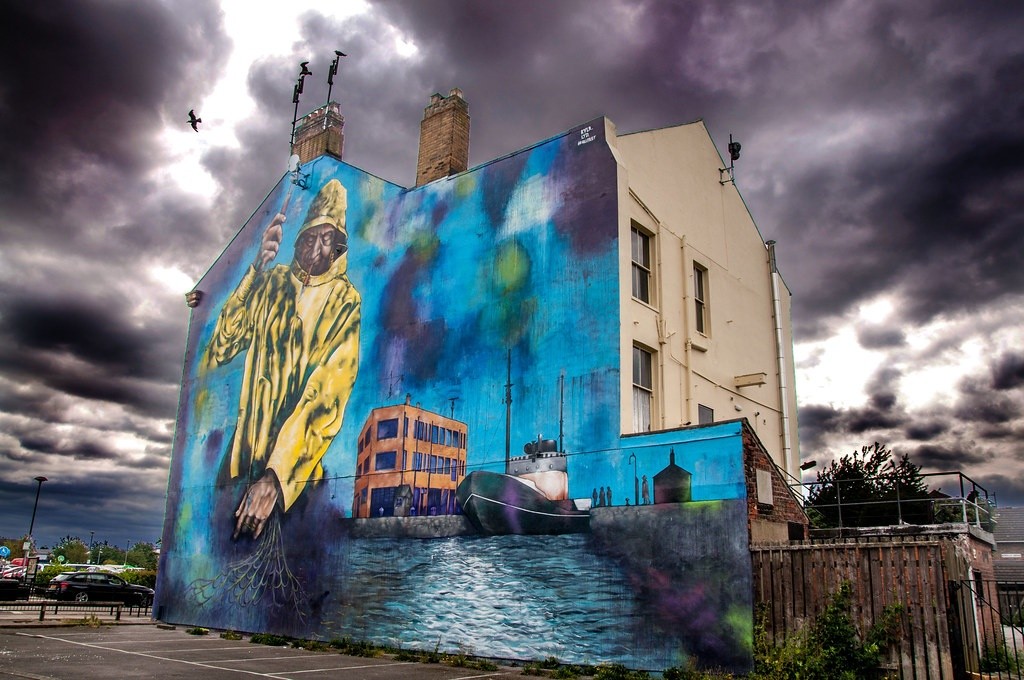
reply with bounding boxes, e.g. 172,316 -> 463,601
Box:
21,475 -> 49,573
97,546 -> 104,566
89,531 -> 96,551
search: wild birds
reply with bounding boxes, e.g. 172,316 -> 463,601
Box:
186,109 -> 202,133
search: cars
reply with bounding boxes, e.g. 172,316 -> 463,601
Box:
45,570 -> 155,608
0,562 -> 134,580
